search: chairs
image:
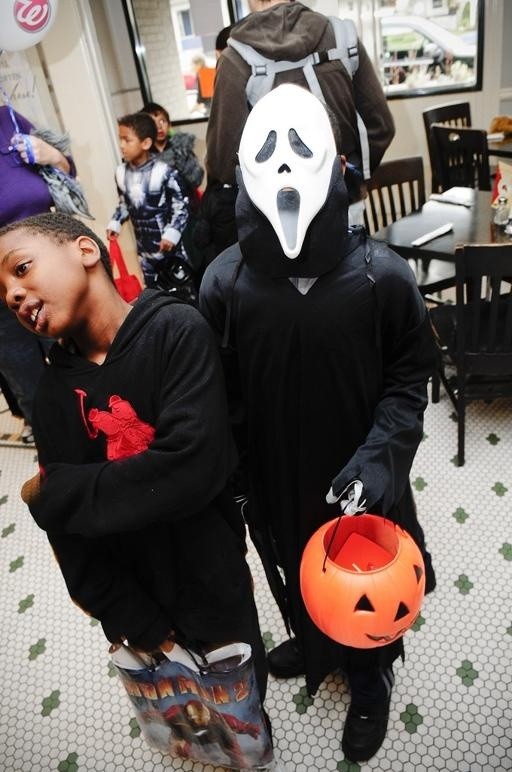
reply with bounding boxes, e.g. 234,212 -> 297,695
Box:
366,157 -> 467,380
428,242 -> 511,466
420,103 -> 491,193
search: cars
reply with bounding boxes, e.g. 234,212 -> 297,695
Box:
377,13 -> 475,86
179,33 -> 205,89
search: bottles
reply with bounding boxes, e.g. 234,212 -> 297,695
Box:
493,197 -> 509,225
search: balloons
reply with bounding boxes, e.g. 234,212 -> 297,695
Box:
0,0 -> 57,54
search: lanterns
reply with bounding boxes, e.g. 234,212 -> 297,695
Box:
299,500 -> 427,652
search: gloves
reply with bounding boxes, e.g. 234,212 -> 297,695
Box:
326,380 -> 427,516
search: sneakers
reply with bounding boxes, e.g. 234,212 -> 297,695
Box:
268,638 -> 304,678
343,698 -> 389,762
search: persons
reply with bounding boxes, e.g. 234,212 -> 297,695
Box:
103,112 -> 198,306
137,101 -> 205,268
213,26 -> 231,60
203,0 -> 396,260
196,82 -> 438,763
0,103 -> 77,444
0,213 -> 277,770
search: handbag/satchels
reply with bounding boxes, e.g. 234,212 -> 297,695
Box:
108,640 -> 275,770
110,236 -> 143,303
38,166 -> 95,221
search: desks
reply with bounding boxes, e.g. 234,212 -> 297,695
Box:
367,184 -> 512,304
